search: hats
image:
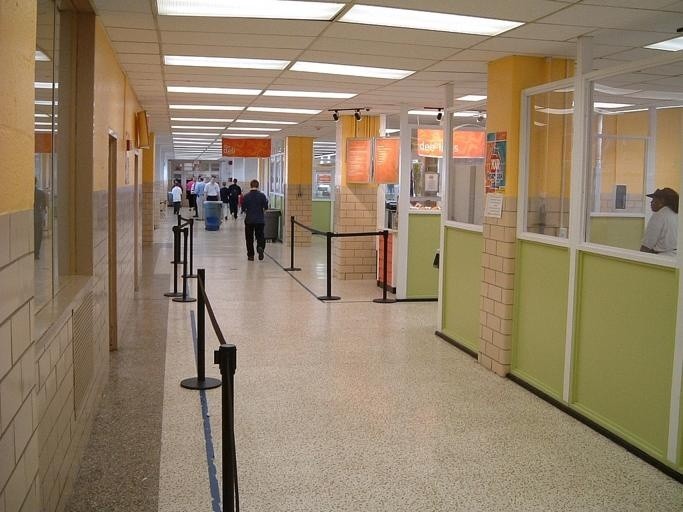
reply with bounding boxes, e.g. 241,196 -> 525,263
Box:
645,188 -> 673,199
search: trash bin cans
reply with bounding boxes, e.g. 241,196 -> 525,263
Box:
263,209 -> 282,243
203,201 -> 224,231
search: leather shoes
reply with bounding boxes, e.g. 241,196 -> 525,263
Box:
248,247 -> 264,261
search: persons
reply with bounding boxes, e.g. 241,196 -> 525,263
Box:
640,188 -> 679,258
33,177 -> 49,259
242,179 -> 268,260
171,175 -> 241,220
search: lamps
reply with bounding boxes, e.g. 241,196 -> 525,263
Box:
424,107 -> 444,121
328,109 -> 364,120
